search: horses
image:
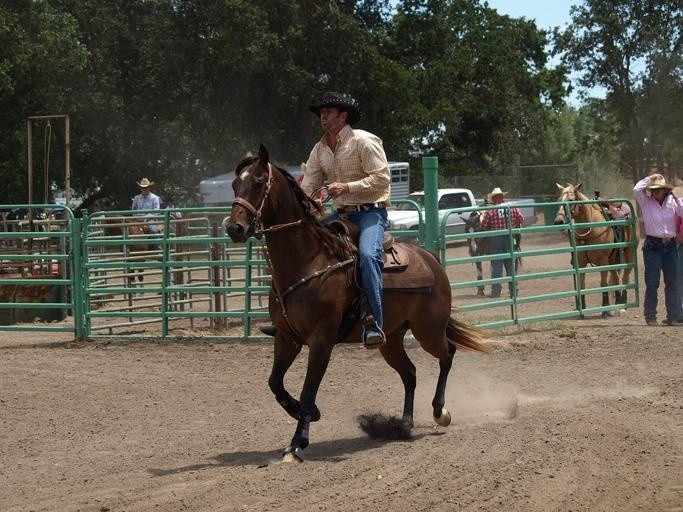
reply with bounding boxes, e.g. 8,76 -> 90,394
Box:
103,212 -> 188,298
554,182 -> 641,320
459,201 -> 523,300
223,141 -> 493,465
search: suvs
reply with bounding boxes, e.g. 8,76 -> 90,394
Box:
6,204 -> 74,234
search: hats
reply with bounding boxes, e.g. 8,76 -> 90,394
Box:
137,177 -> 153,188
648,174 -> 673,190
310,91 -> 361,126
487,187 -> 507,199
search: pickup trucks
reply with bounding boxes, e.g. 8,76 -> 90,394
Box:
384,188 -> 538,247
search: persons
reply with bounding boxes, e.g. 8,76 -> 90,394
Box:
477,187 -> 525,297
632,174 -> 683,327
259,92 -> 391,347
131,178 -> 165,256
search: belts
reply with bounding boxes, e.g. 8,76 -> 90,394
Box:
343,202 -> 384,213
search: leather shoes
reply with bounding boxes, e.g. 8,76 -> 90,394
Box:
366,332 -> 383,346
260,325 -> 276,335
